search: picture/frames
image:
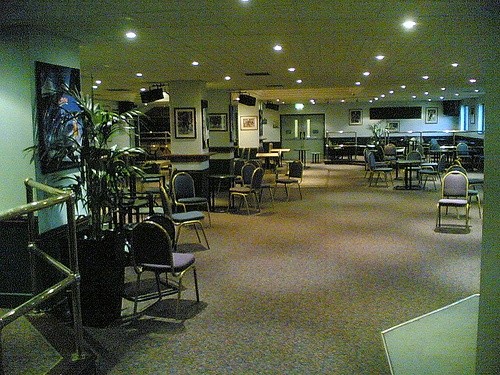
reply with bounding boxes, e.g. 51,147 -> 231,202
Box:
388,121 -> 400,130
35,62 -> 84,175
240,116 -> 258,130
348,109 -> 363,125
206,113 -> 227,132
426,107 -> 439,125
174,108 -> 196,139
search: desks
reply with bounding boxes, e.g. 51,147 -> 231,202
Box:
207,174 -> 237,212
256,153 -> 279,170
395,159 -> 421,190
270,148 -> 290,167
294,148 -> 310,169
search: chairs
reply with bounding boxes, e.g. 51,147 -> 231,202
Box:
122,138 -> 485,318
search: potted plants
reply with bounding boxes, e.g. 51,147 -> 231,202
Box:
22,82 -> 152,326
